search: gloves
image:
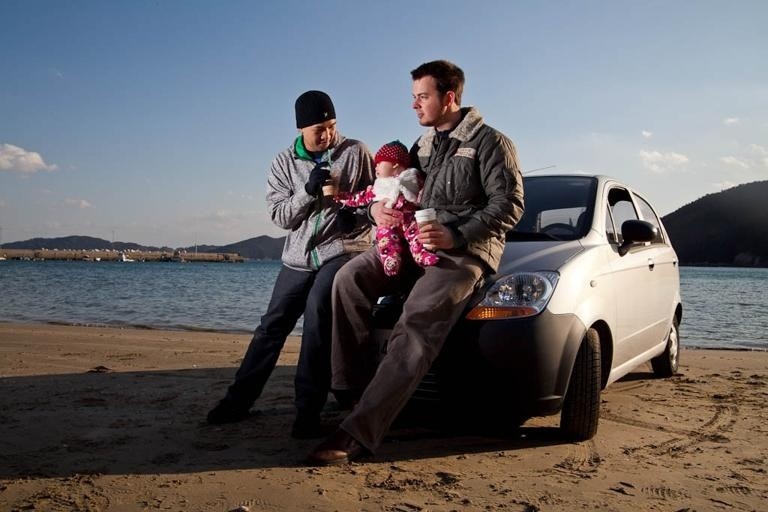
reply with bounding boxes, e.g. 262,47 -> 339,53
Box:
305,161 -> 332,199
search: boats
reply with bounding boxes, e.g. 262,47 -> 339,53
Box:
0,248 -> 246,264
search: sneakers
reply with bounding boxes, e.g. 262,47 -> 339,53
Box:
292,407 -> 321,436
205,400 -> 251,422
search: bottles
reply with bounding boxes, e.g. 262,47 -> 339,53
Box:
320,165 -> 337,205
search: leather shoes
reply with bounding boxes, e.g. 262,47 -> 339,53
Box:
307,428 -> 364,466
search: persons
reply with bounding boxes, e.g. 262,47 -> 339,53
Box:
331,138 -> 440,275
206,89 -> 374,439
307,60 -> 526,462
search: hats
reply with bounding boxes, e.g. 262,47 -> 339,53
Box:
375,141 -> 409,167
295,90 -> 336,130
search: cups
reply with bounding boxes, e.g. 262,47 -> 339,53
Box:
414,208 -> 439,248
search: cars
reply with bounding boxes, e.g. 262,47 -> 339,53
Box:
361,165 -> 686,445
